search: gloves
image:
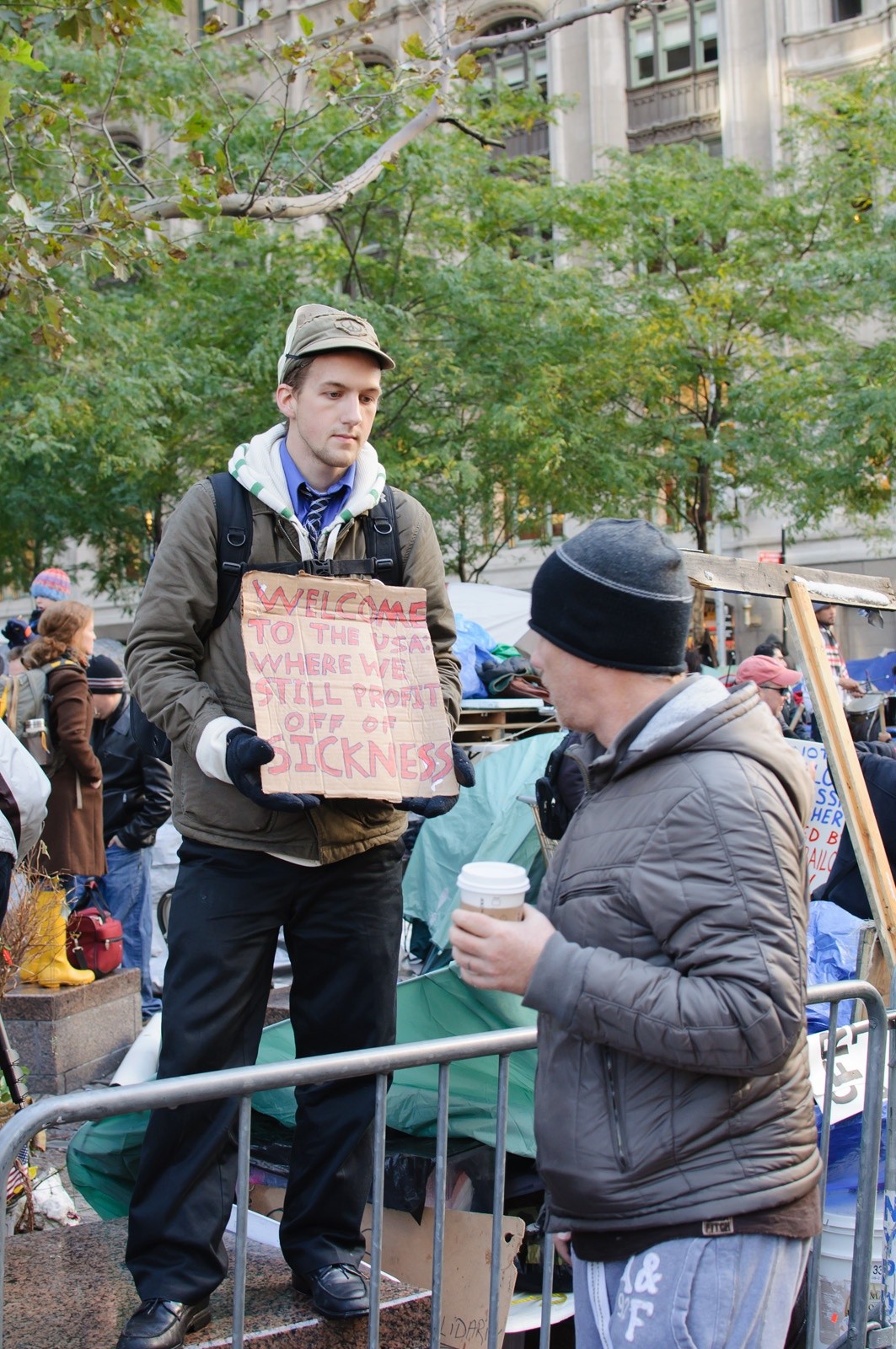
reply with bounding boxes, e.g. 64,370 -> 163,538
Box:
1,625 -> 24,647
7,618 -> 35,643
226,728 -> 320,809
392,742 -> 475,819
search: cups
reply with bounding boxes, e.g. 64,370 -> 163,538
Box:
456,861 -> 531,922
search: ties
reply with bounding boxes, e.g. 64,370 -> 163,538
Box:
299,484 -> 337,551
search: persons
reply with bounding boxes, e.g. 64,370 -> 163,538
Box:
118,304 -> 475,1347
676,601 -> 896,920
452,515 -> 823,1349
0,561 -> 172,1028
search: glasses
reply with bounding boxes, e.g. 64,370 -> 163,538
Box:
760,684 -> 789,696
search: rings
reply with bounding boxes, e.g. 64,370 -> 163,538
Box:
466,956 -> 473,972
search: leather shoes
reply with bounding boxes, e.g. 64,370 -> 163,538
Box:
290,1262 -> 370,1320
118,1295 -> 212,1349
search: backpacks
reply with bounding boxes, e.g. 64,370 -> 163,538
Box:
0,661 -> 85,780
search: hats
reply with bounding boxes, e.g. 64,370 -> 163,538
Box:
528,519 -> 693,673
86,654 -> 123,694
278,304 -> 395,383
30,568 -> 71,603
737,654 -> 803,688
812,602 -> 830,612
